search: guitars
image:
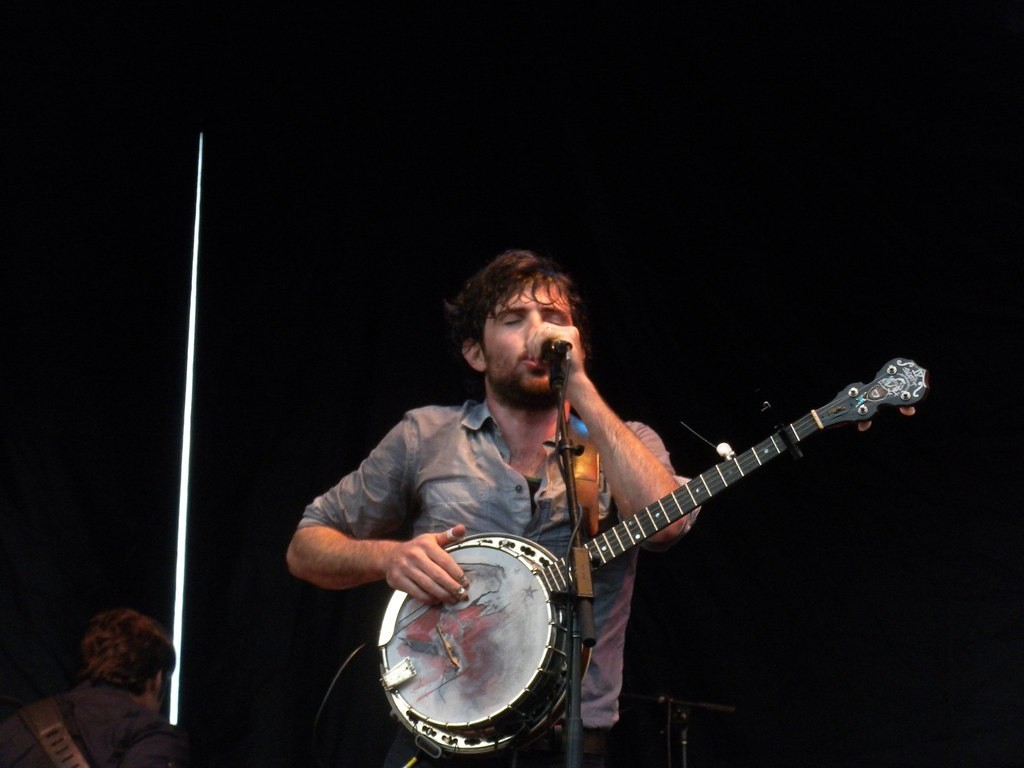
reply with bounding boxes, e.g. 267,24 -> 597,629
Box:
374,353 -> 936,763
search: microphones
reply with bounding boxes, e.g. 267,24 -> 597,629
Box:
541,340 -> 573,361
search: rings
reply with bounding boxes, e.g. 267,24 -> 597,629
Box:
446,528 -> 457,544
546,325 -> 552,333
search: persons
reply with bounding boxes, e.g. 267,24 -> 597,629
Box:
0,608 -> 189,768
284,243 -> 702,768
